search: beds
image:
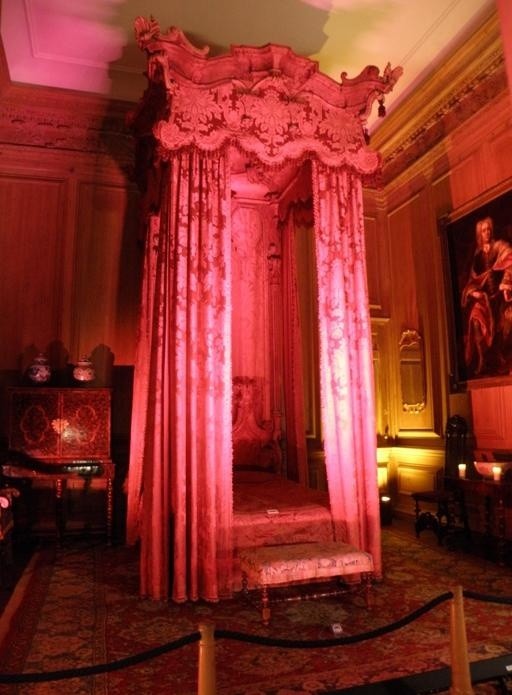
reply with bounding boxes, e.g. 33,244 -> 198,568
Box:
123,14 -> 404,604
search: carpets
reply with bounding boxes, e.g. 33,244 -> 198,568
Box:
0,526 -> 511,693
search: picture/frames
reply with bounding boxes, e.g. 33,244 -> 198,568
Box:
433,173 -> 511,396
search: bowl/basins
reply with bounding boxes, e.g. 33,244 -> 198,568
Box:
473,459 -> 512,479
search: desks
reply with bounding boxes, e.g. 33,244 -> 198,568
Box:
442,475 -> 511,568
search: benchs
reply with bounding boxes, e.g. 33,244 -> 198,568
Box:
238,541 -> 376,628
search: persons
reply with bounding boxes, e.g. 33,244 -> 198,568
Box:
460,215 -> 512,376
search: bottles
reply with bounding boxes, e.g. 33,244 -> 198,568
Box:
28,354 -> 52,385
72,358 -> 96,384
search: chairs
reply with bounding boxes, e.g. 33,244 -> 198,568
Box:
408,412 -> 471,551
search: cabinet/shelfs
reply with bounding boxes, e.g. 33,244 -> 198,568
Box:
1,386 -> 115,549
